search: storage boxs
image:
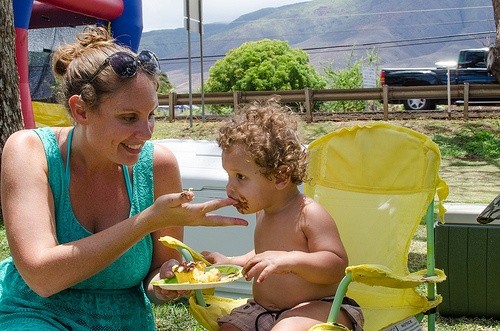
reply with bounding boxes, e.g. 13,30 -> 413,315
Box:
432,203 -> 499,319
143,138 -> 259,296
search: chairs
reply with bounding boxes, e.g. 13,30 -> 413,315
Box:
158,119 -> 447,331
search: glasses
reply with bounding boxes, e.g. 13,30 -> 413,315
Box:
77,51 -> 160,95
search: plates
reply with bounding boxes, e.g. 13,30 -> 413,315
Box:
149,264 -> 244,291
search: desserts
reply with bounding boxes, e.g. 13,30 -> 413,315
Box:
171,259 -> 222,284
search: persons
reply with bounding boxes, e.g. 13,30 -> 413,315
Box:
0,26 -> 250,331
216,94 -> 364,331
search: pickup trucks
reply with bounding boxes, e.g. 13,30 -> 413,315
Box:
378,48 -> 500,111
